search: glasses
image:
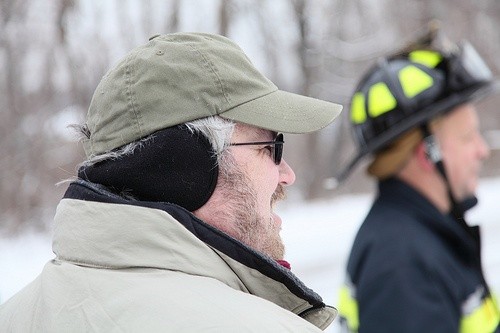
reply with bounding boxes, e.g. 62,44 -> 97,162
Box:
216,133 -> 284,165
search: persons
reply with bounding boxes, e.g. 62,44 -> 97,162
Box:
0,32 -> 351,333
338,21 -> 500,333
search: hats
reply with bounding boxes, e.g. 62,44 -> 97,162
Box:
79,31 -> 344,160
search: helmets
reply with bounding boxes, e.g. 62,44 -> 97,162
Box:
337,34 -> 496,184
